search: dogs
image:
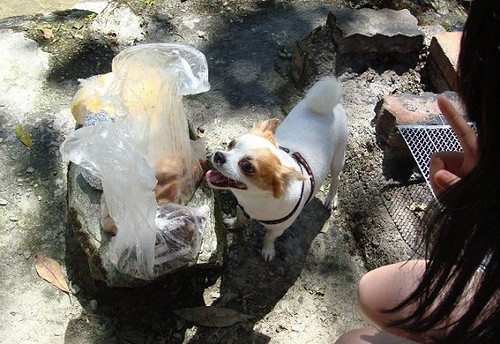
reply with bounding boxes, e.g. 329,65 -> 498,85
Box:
204,75 -> 348,263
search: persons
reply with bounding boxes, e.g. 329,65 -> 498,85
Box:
335,0 -> 500,344
